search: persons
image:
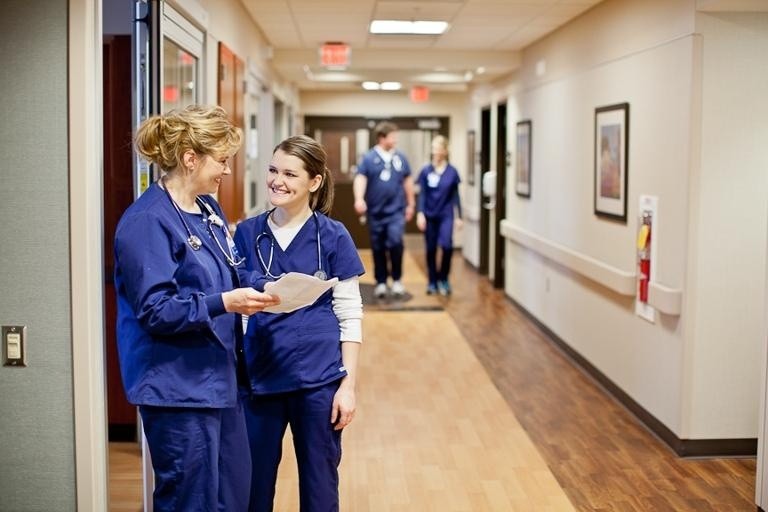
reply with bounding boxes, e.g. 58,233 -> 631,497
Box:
233,136 -> 364,511
414,135 -> 464,295
351,119 -> 416,298
112,105 -> 282,511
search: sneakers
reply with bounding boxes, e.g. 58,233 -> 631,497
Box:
391,281 -> 404,296
374,282 -> 387,298
436,278 -> 450,297
426,283 -> 437,294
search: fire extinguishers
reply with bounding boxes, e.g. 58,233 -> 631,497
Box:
638,210 -> 651,303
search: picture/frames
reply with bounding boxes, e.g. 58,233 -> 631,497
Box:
514,118 -> 534,199
590,101 -> 631,222
464,129 -> 475,187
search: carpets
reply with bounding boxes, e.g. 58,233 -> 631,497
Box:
261,241 -> 579,512
